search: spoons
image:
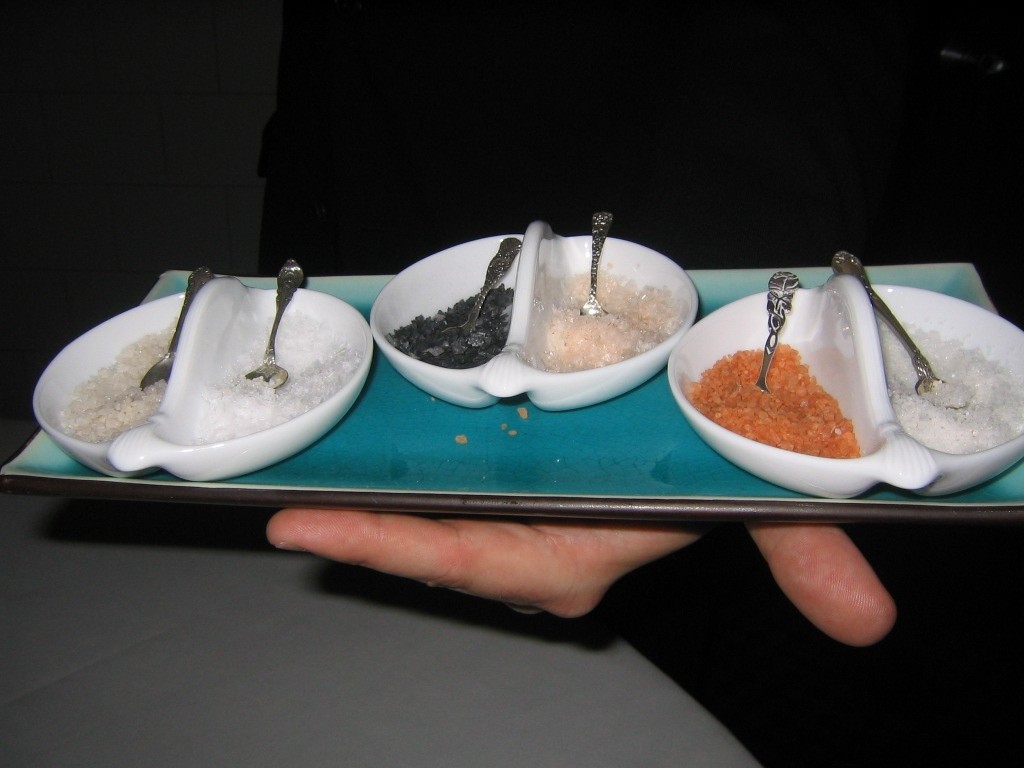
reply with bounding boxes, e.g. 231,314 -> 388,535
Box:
831,250 -> 972,408
139,267 -> 216,392
440,237 -> 522,336
243,259 -> 304,388
579,211 -> 612,318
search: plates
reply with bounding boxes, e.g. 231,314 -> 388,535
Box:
30,277 -> 375,483
666,286 -> 1024,499
368,217 -> 699,414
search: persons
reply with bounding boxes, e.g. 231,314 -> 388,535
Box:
255,0 -> 1024,768
